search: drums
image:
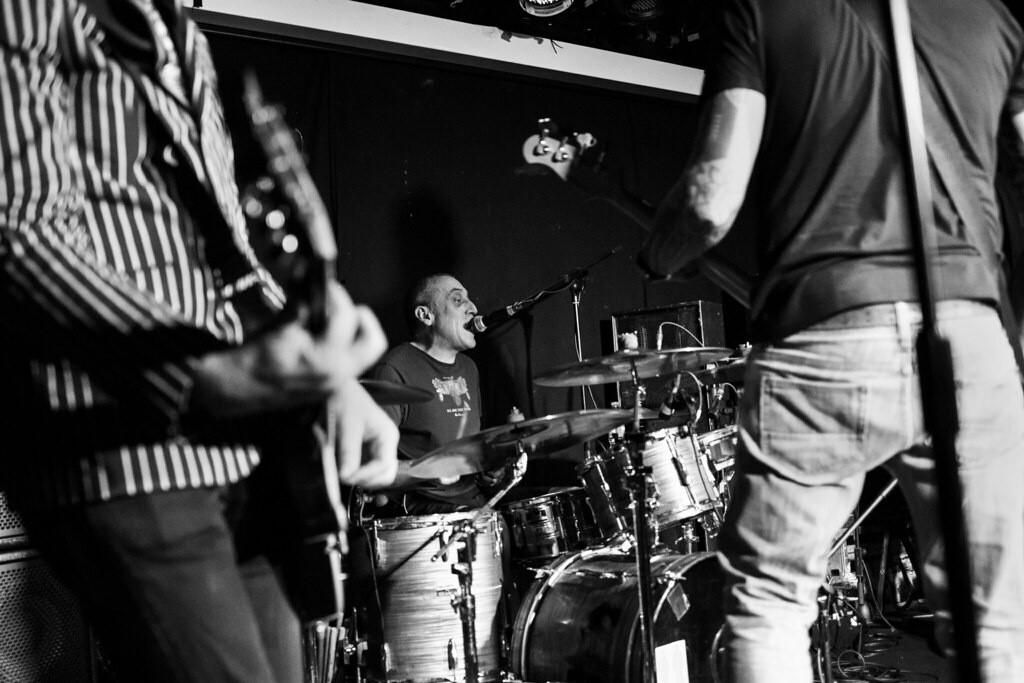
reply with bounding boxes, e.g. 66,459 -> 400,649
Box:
507,484 -> 609,563
697,424 -> 739,472
367,505 -> 511,683
510,533 -> 729,683
574,424 -> 726,536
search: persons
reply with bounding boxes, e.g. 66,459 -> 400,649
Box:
0,0 -> 400,683
364,275 -> 527,518
636,0 -> 1024,683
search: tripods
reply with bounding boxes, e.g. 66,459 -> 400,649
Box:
851,503 -> 916,660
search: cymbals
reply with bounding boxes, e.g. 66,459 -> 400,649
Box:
358,378 -> 436,408
408,408 -> 635,481
530,344 -> 734,389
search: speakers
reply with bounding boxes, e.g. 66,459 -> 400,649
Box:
612,300 -> 726,410
0,550 -> 97,683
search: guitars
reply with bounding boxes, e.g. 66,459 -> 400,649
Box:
520,114 -> 755,313
229,63 -> 369,627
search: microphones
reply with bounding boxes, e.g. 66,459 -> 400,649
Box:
659,373 -> 682,420
471,299 -> 535,333
707,385 -> 725,420
